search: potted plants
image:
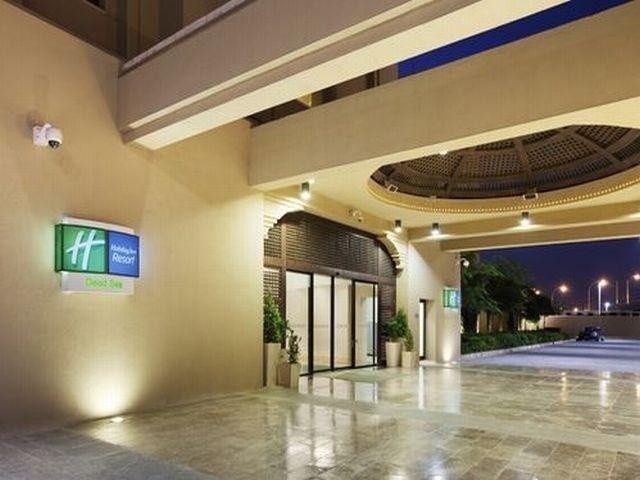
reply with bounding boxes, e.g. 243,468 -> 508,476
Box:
385,307 -> 419,368
262,285 -> 301,389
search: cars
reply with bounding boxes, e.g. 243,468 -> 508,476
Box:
576,326 -> 601,342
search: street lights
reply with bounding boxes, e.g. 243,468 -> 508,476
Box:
588,279 -> 605,314
626,274 -> 640,302
550,286 -> 566,301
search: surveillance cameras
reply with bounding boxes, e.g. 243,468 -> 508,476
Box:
32,125 -> 62,150
357,215 -> 363,224
463,260 -> 469,267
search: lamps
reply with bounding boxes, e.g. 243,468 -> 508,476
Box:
300,181 -> 530,237
